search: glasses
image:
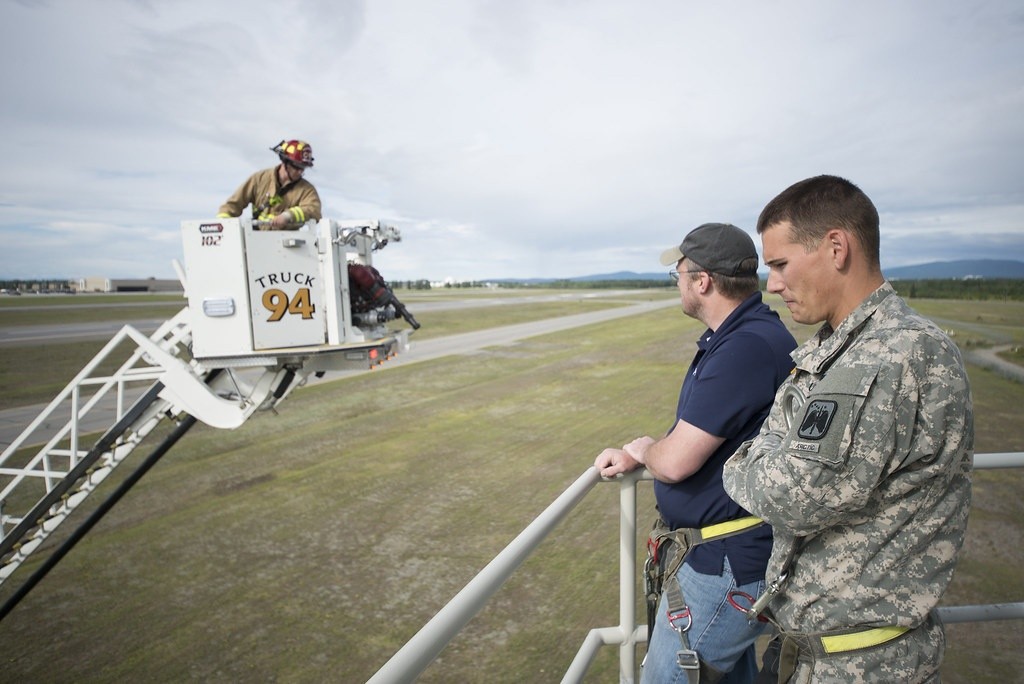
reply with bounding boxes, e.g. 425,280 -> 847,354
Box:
669,269 -> 708,280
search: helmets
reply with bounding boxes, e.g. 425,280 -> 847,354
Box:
660,224 -> 760,279
278,139 -> 313,171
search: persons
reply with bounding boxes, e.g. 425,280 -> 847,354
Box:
218,140 -> 321,231
722,175 -> 976,684
594,223 -> 798,684
